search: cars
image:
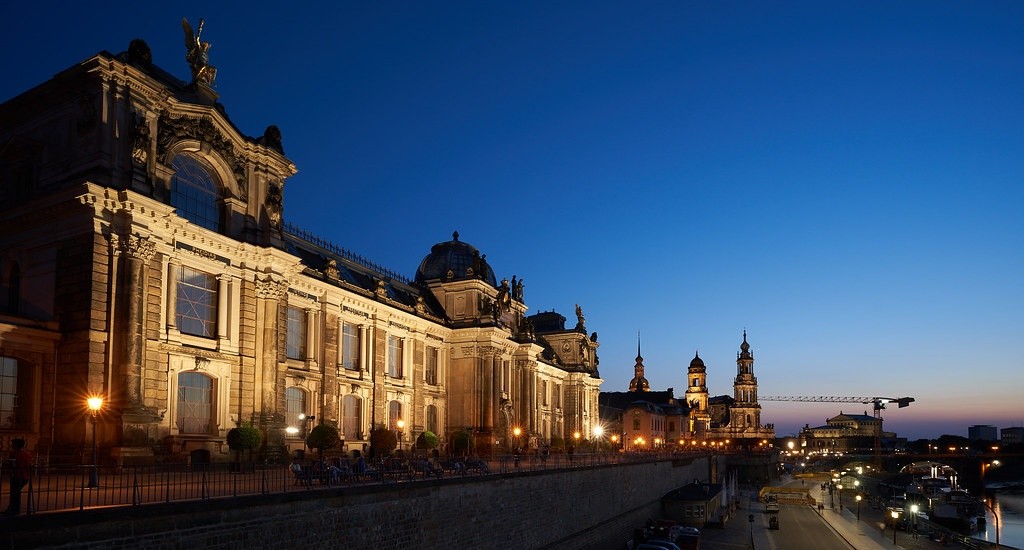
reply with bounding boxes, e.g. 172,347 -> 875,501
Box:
637,544 -> 668,550
649,540 -> 681,550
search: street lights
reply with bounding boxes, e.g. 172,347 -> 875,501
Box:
286,412 -> 308,491
398,421 -> 404,459
891,511 -> 899,545
86,396 -> 105,487
856,495 -> 862,520
514,427 -> 522,454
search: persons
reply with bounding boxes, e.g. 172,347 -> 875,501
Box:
575,303 -> 586,324
818,503 -> 824,515
3,439 -> 32,516
289,456 -> 371,487
567,445 -> 574,462
190,21 -> 219,82
424,454 -> 473,476
513,445 -> 523,467
474,254 -> 536,334
541,444 -> 550,463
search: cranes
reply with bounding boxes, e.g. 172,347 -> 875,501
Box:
757,395 -> 916,476
